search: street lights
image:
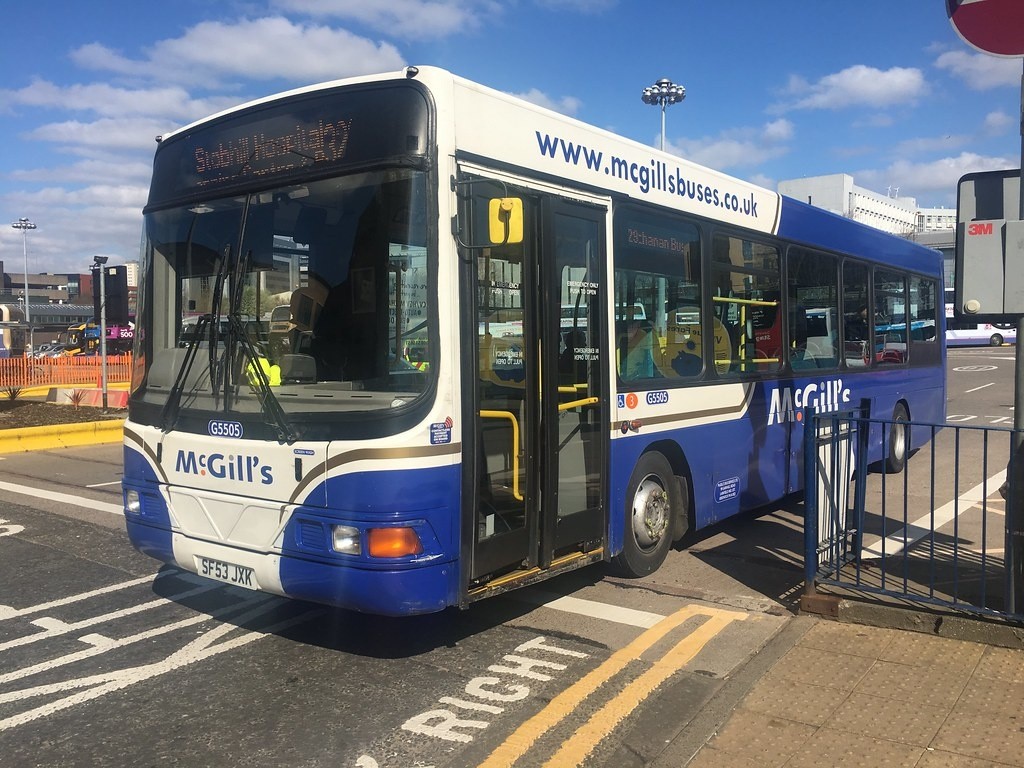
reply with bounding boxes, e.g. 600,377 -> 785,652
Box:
11,217 -> 36,323
641,76 -> 687,152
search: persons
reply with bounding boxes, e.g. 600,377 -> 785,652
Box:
845,304 -> 869,341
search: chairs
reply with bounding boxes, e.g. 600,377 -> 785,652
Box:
754,340 -> 836,371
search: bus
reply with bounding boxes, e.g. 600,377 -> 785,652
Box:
0,304 -> 24,357
477,303 -> 646,341
888,288 -> 1016,349
121,64 -> 948,618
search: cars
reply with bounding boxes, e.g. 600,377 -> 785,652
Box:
27,316 -> 135,359
